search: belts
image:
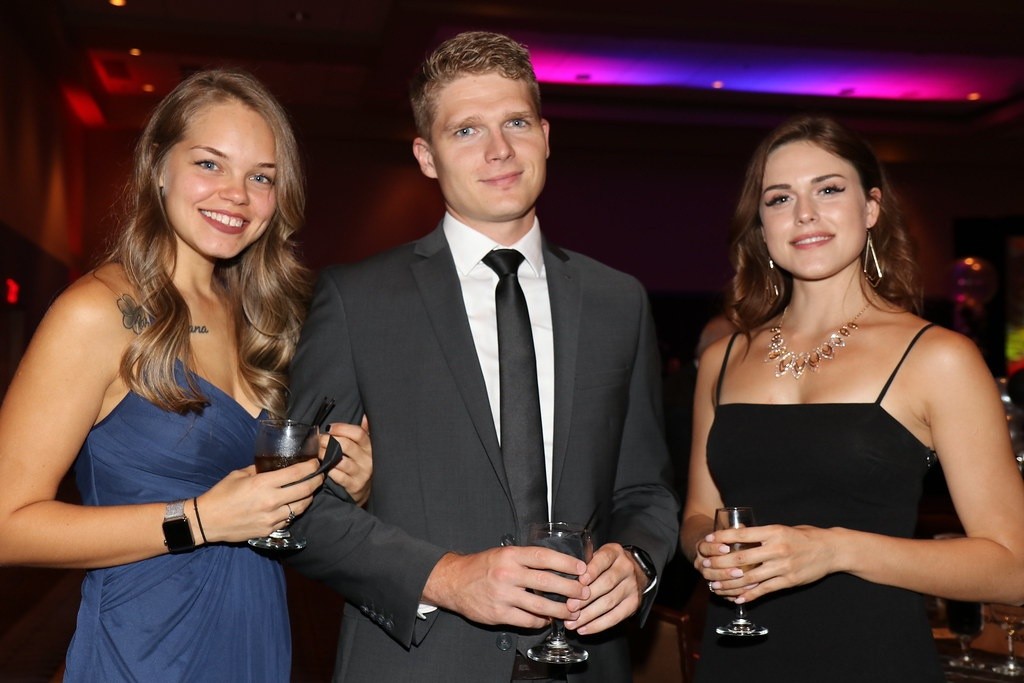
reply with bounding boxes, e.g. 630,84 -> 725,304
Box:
511,656 -> 564,679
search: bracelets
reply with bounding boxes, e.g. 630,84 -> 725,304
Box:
194,497 -> 208,543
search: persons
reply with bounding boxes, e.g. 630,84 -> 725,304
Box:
285,32 -> 680,683
0,72 -> 373,683
681,115 -> 1024,683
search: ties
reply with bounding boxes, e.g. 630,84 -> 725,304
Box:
482,249 -> 552,661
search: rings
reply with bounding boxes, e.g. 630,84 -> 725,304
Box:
708,581 -> 714,592
287,504 -> 295,522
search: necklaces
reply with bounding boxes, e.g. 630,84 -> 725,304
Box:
761,295 -> 877,379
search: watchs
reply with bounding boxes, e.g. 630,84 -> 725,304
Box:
162,499 -> 195,552
624,545 -> 657,581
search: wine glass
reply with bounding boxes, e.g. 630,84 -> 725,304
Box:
948,605 -> 987,668
713,507 -> 769,637
991,604 -> 1024,677
247,418 -> 320,551
527,522 -> 588,664
